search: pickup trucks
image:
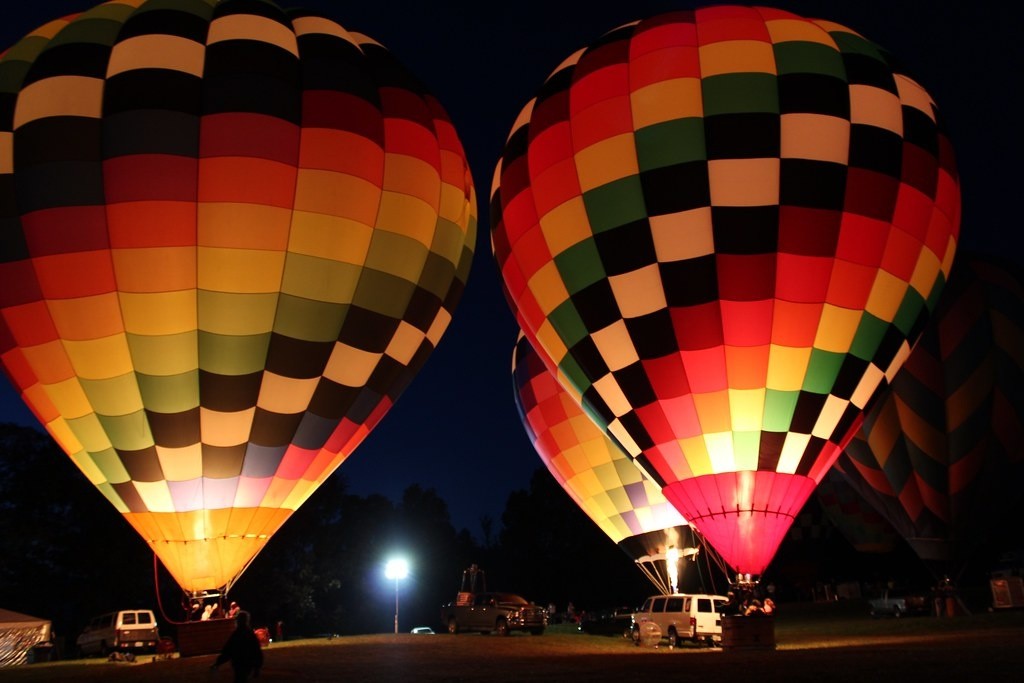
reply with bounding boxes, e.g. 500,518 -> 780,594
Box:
871,587 -> 929,619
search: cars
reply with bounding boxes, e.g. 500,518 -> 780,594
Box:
613,609 -> 632,622
441,592 -> 548,636
410,627 -> 436,635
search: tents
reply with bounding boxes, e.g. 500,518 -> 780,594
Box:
0,609 -> 51,668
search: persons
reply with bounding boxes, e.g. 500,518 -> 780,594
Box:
276,621 -> 282,641
980,569 -> 992,612
210,613 -> 261,683
548,602 -> 556,625
719,591 -> 775,617
931,576 -> 955,618
566,602 -> 574,624
185,602 -> 240,621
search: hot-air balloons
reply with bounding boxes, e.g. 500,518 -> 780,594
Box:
1,0 -> 480,656
485,0 -> 1024,651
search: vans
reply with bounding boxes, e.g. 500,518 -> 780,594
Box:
75,609 -> 161,655
630,593 -> 729,647
